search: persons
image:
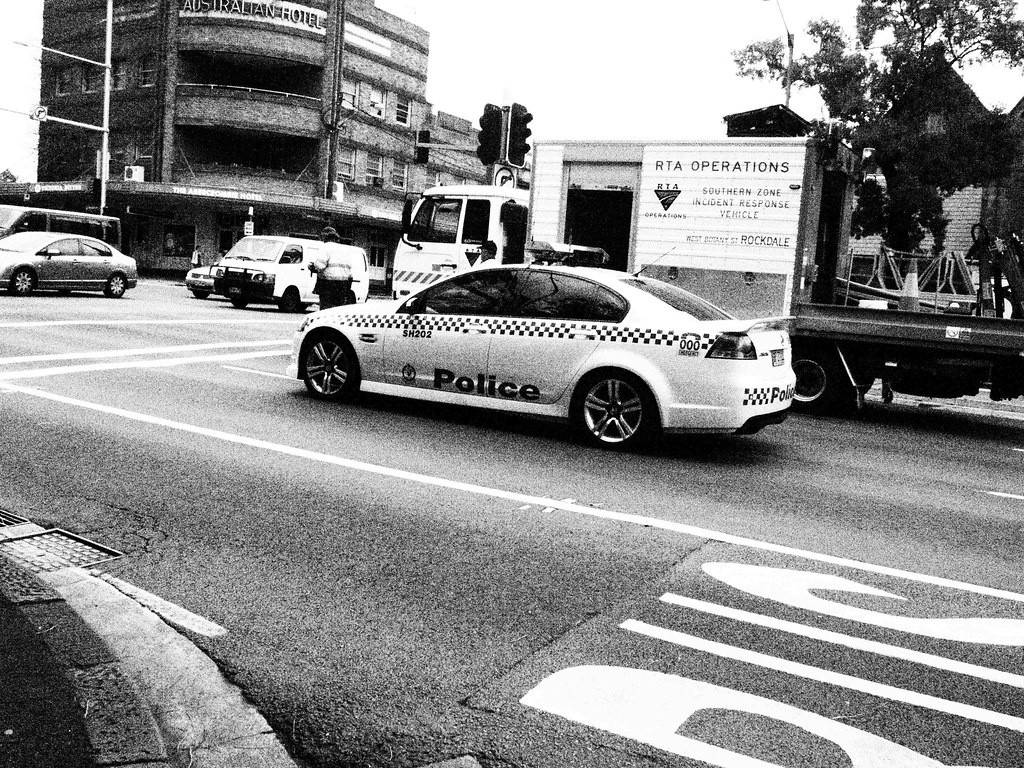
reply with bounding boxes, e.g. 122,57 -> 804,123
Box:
308,227 -> 352,308
192,245 -> 202,268
475,241 -> 499,267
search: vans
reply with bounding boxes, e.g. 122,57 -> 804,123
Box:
0,203 -> 122,253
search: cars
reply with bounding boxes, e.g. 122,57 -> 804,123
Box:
287,261 -> 797,450
1,231 -> 139,298
212,236 -> 358,314
183,265 -> 219,300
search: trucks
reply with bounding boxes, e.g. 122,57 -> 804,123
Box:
383,132 -> 1024,418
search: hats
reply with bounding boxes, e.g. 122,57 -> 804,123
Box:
477,241 -> 497,253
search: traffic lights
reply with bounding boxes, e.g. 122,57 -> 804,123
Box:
509,104 -> 533,169
476,101 -> 502,166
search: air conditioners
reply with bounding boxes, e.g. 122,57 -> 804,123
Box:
323,179 -> 344,202
125,165 -> 146,182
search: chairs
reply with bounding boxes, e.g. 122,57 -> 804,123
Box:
505,294 -> 540,317
562,296 -> 593,321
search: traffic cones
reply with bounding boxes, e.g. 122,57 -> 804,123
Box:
896,258 -> 921,313
